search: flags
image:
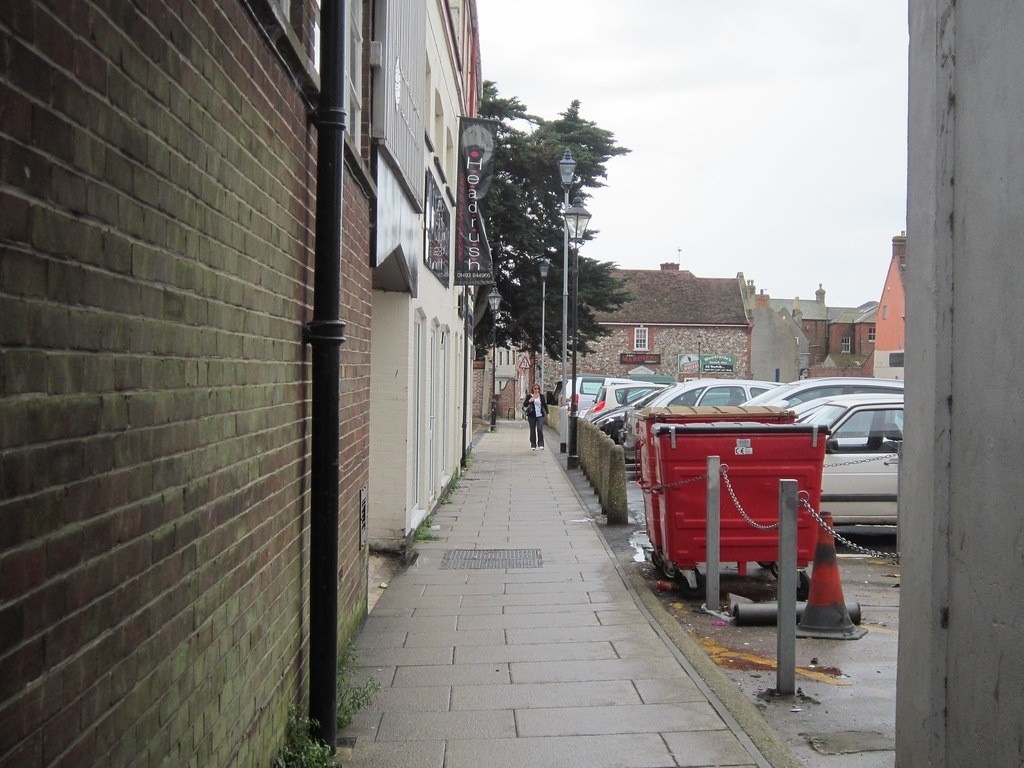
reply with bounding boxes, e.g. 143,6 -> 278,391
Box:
453,115 -> 499,286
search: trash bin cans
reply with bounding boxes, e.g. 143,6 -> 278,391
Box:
632,404 -> 799,569
650,422 -> 833,579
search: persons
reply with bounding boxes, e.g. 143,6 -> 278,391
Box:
523,383 -> 549,451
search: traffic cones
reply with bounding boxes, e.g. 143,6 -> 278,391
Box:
796,511 -> 869,640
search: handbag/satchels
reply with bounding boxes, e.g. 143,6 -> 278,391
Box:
521,393 -> 535,413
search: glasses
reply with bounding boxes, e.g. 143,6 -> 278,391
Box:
533,388 -> 539,390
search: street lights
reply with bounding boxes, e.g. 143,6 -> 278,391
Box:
696,332 -> 702,380
559,146 -> 578,454
562,195 -> 594,472
538,255 -> 550,395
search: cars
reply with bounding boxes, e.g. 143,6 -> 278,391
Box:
562,369 -> 905,536
